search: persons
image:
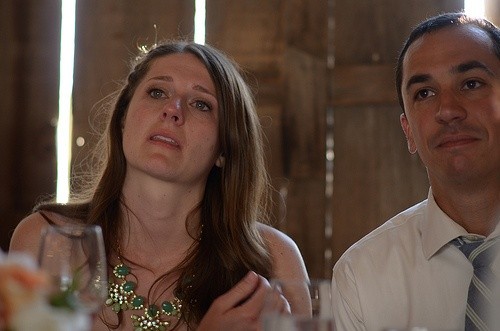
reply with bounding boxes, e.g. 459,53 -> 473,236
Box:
330,13 -> 500,331
8,41 -> 312,331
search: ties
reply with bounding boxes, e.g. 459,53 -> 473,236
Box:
452,236 -> 500,330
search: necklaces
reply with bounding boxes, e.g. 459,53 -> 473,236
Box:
105,224 -> 204,331
